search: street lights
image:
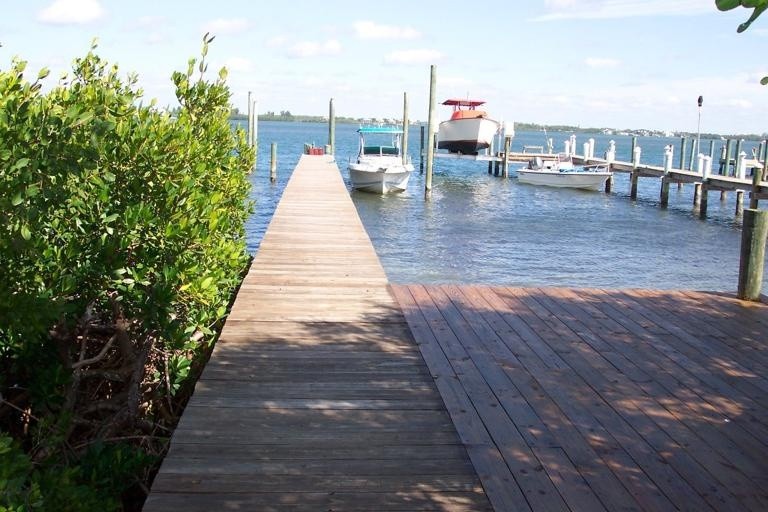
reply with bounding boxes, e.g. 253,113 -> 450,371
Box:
695,94 -> 705,175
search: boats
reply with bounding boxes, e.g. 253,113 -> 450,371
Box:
433,98 -> 501,156
731,153 -> 766,179
515,154 -> 613,191
347,123 -> 415,195
